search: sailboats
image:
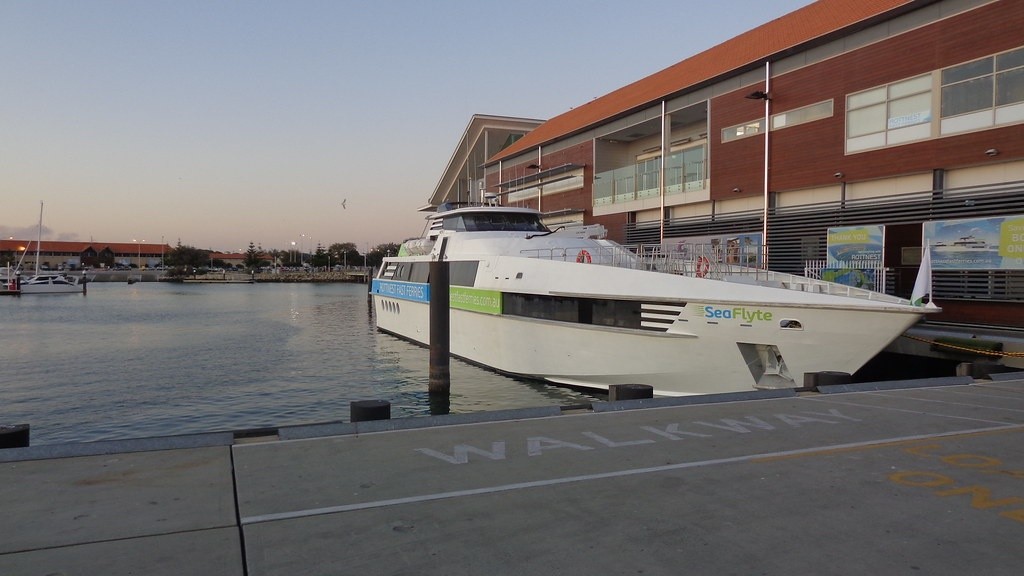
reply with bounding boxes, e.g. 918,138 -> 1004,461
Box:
3,203 -> 83,293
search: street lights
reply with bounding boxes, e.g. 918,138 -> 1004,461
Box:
133,240 -> 145,268
162,236 -> 164,271
344,251 -> 346,267
387,248 -> 390,266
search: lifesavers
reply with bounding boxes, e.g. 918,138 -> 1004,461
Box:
576,250 -> 594,265
695,255 -> 709,279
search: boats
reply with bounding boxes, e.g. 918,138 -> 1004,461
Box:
929,236 -> 999,256
368,177 -> 944,397
